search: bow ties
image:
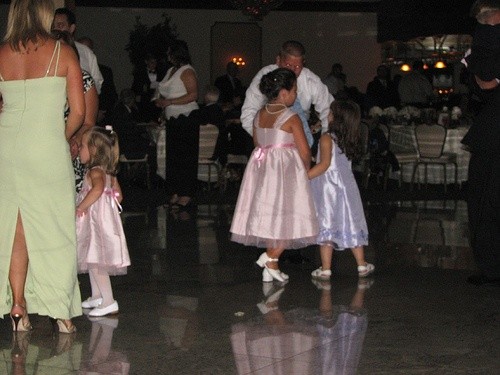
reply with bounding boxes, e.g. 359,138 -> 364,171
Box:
149,70 -> 156,74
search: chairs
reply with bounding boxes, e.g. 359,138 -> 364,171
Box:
360,119 -> 458,227
119,153 -> 150,191
198,124 -> 250,214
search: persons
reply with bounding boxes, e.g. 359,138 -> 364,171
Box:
0,1 -> 131,334
307,98 -> 375,281
366,64 -> 401,111
131,40 -> 243,210
459,1 -> 500,284
240,40 -> 336,169
408,61 -> 431,108
230,68 -> 319,282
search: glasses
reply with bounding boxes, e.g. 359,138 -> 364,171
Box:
475,10 -> 497,20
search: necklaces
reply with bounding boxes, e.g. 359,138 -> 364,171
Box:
264,103 -> 287,115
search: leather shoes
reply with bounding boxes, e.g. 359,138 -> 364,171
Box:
89,301 -> 119,316
88,316 -> 119,328
81,297 -> 103,307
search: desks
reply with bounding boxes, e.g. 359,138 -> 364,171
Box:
147,121 -> 219,182
389,123 -> 469,189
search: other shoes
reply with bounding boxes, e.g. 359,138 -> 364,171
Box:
163,199 -> 191,211
280,254 -> 310,265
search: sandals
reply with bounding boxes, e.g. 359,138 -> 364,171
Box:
311,279 -> 330,290
358,279 -> 374,289
358,263 -> 374,277
311,266 -> 331,279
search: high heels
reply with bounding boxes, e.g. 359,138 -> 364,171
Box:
264,284 -> 273,297
256,288 -> 284,314
262,268 -> 288,281
11,304 -> 31,331
10,332 -> 31,361
48,316 -> 76,338
50,333 -> 72,359
257,252 -> 284,283
